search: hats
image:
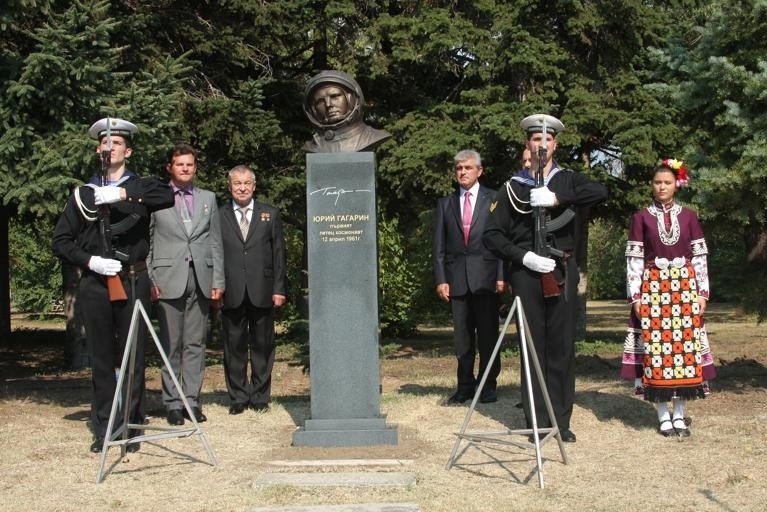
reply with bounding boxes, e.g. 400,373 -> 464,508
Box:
520,114 -> 566,137
88,117 -> 138,141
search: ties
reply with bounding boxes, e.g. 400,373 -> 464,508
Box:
178,190 -> 191,235
237,207 -> 250,241
463,192 -> 473,245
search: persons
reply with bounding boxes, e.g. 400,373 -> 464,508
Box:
619,159 -> 718,436
298,69 -> 396,151
51,119 -> 174,449
500,144 -> 532,411
432,150 -> 506,402
144,145 -> 227,426
485,112 -> 605,442
217,164 -> 287,418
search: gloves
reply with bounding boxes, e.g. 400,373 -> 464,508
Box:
530,185 -> 556,206
94,186 -> 121,205
523,250 -> 556,274
88,256 -> 122,277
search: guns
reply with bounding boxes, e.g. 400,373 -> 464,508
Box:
95,115 -> 141,301
534,118 -> 575,298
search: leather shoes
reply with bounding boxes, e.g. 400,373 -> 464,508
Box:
672,419 -> 691,437
554,429 -> 576,443
90,440 -> 110,452
167,410 -> 184,425
127,443 -> 139,452
230,403 -> 248,414
183,407 -> 207,422
659,420 -> 676,437
529,433 -> 548,443
449,392 -> 474,404
480,391 -> 496,403
254,404 -> 269,413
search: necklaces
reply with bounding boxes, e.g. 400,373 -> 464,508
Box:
651,200 -> 679,238
